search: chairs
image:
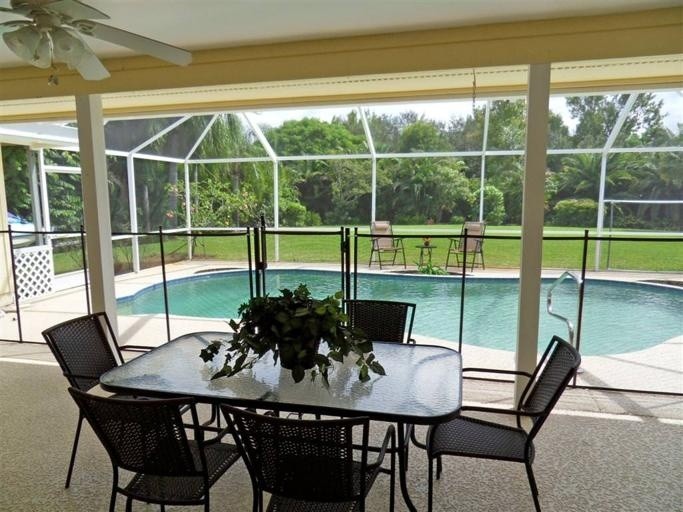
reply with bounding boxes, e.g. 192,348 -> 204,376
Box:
446,221 -> 486,273
41,312 -> 126,489
369,221 -> 406,270
220,401 -> 396,512
342,299 -> 416,346
68,387 -> 242,512
427,336 -> 581,512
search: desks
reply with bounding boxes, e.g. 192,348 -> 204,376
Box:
101,331 -> 463,512
416,245 -> 437,269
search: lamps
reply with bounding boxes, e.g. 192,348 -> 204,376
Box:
0,14 -> 84,86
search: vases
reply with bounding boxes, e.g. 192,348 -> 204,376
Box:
424,242 -> 430,245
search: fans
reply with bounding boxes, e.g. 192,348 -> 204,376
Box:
0,0 -> 193,81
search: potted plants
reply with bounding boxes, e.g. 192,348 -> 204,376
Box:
198,284 -> 385,390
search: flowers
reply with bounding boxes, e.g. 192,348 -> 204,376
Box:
423,219 -> 433,242
